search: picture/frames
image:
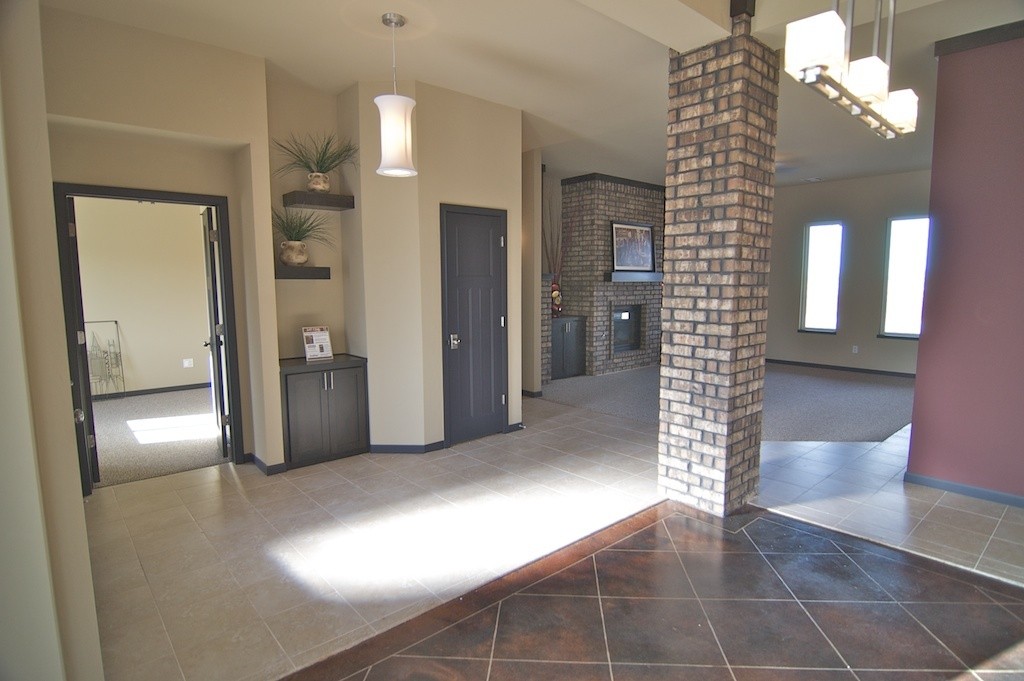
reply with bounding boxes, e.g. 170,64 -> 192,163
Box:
611,220 -> 657,273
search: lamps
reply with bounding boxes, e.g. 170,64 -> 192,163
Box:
372,14 -> 419,177
784,1 -> 920,139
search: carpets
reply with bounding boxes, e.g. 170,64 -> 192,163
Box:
536,361 -> 915,443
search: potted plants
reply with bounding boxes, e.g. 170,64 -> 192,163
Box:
274,129 -> 360,193
270,208 -> 338,267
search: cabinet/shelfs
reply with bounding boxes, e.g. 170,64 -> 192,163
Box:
552,315 -> 587,380
280,353 -> 369,467
274,189 -> 355,279
